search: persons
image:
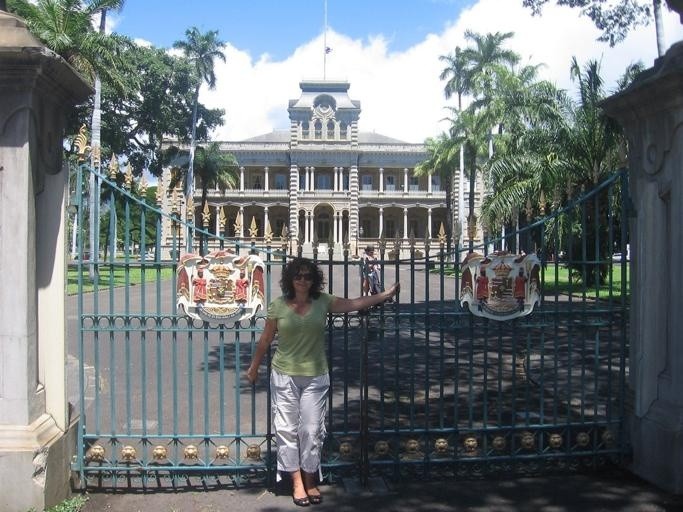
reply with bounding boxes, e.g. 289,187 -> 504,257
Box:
235,268 -> 250,307
361,245 -> 380,297
246,258 -> 401,507
514,268 -> 527,313
476,266 -> 489,312
192,267 -> 206,307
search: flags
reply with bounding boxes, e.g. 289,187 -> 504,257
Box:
325,47 -> 332,54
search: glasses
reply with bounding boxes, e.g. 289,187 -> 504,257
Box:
293,273 -> 313,281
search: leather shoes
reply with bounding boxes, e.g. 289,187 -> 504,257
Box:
292,485 -> 310,506
309,485 -> 321,504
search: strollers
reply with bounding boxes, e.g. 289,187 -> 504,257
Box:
368,269 -> 398,312
